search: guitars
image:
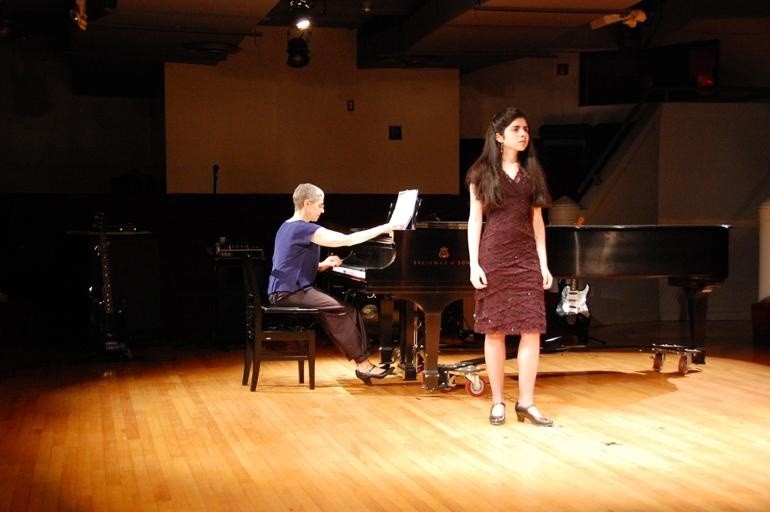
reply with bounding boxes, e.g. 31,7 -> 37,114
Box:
553,276 -> 591,335
88,212 -> 132,363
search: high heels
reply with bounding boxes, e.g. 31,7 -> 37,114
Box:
356,364 -> 390,383
515,401 -> 553,426
360,365 -> 394,386
489,402 -> 506,425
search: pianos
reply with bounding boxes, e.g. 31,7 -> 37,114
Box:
332,221 -> 731,391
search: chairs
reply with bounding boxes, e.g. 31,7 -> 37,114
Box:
239,250 -> 319,393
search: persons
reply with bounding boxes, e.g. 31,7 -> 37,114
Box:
465,107 -> 552,427
268,181 -> 395,385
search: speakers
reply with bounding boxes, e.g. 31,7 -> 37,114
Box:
214,257 -> 269,345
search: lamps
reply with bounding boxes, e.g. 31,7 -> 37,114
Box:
286,35 -> 311,67
